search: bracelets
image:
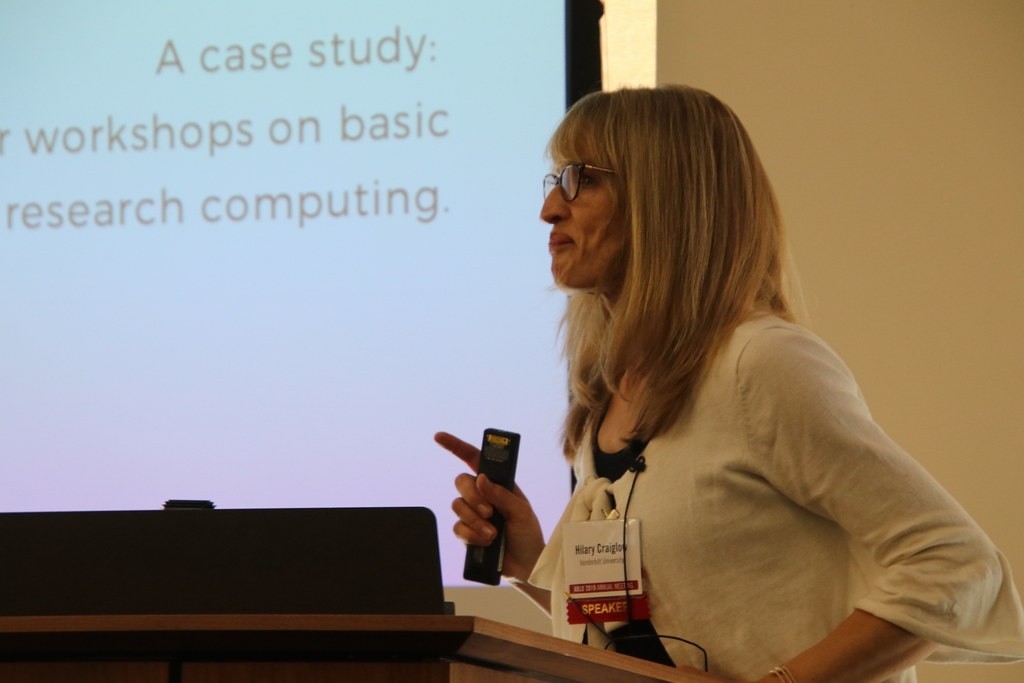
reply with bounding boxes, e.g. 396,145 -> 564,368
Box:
770,664 -> 797,682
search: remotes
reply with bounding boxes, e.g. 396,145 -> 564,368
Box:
463,428 -> 521,586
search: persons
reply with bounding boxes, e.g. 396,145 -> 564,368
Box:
434,82 -> 1024,683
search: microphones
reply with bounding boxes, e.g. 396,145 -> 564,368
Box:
630,454 -> 647,473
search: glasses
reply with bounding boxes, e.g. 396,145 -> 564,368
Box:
543,163 -> 617,202
